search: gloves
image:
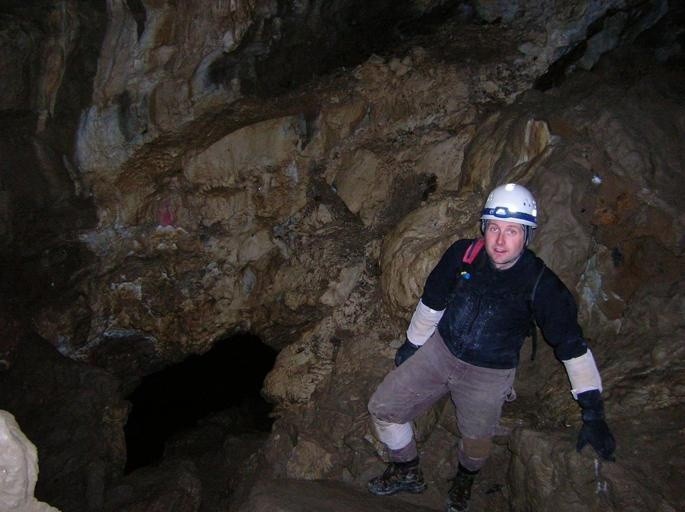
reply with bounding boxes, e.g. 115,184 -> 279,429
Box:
395,336 -> 422,367
576,389 -> 618,463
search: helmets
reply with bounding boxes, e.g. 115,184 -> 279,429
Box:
480,183 -> 538,229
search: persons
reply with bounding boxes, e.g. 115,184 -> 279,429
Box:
367,183 -> 616,512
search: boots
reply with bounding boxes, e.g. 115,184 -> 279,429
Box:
367,455 -> 428,496
447,461 -> 482,512
